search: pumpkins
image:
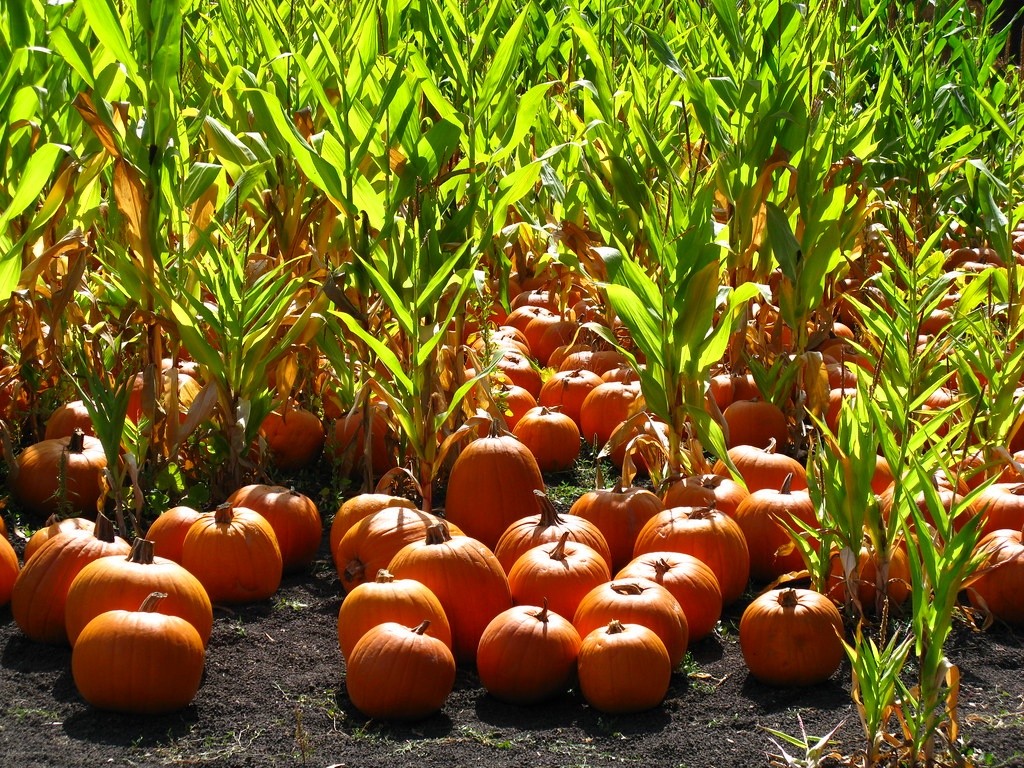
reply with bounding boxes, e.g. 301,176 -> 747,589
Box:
0,215 -> 1024,712
